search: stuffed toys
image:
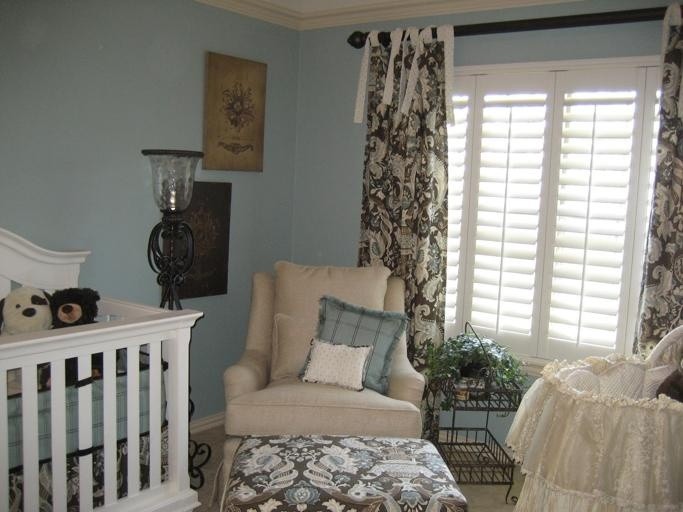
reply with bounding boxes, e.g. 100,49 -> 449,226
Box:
0,283 -> 118,387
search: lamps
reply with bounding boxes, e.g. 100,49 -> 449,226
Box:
141,147 -> 206,312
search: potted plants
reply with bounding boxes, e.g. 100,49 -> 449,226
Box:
423,333 -> 526,415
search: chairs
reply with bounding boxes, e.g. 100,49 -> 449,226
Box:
222,259 -> 428,438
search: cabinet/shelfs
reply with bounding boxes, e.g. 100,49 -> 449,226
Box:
433,373 -> 525,509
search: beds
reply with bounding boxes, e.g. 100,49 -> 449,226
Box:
0,232 -> 202,511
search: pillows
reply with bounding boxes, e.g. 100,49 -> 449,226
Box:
269,296 -> 408,392
